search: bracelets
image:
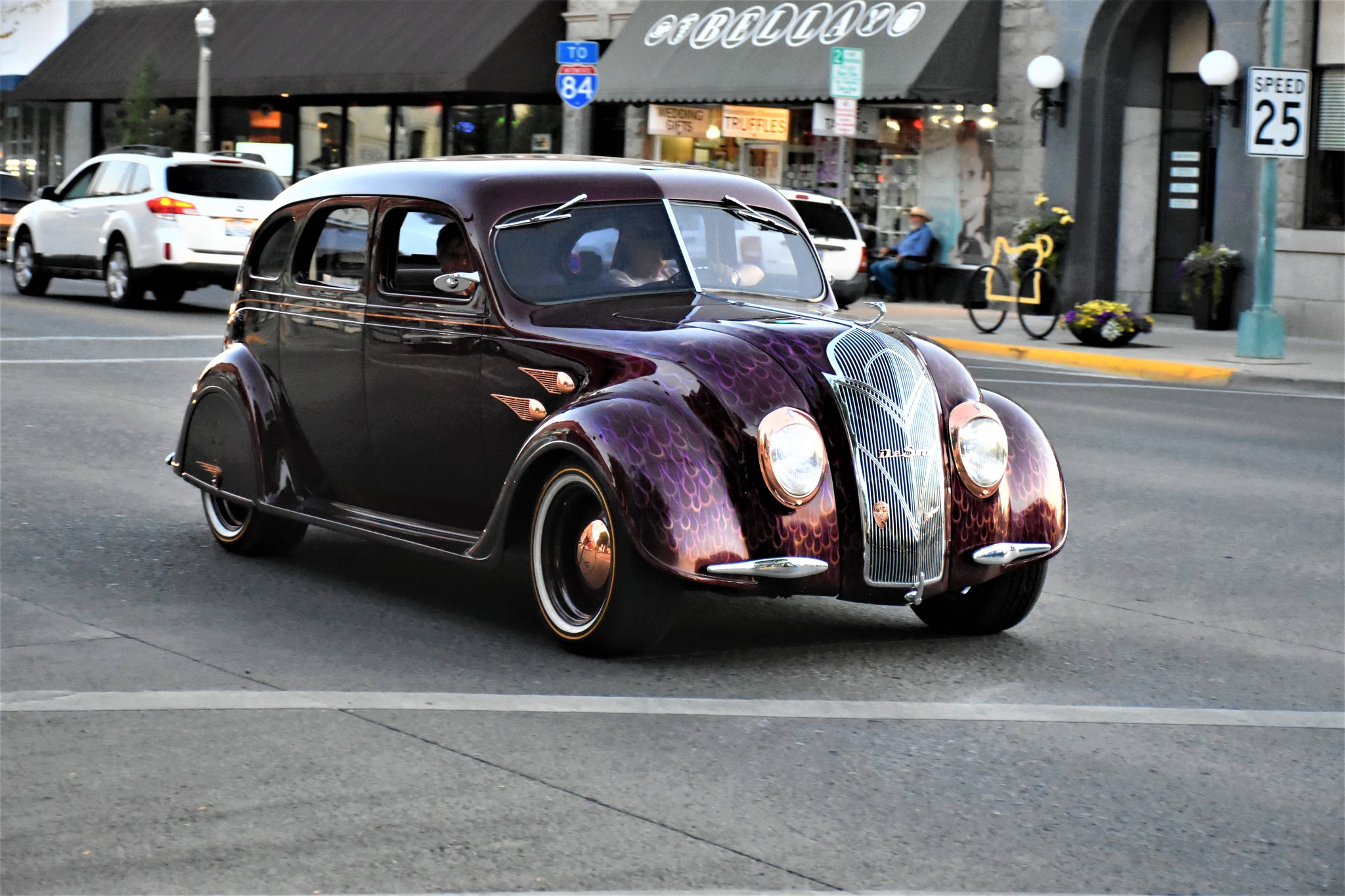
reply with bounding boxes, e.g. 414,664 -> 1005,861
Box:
733,270 -> 741,286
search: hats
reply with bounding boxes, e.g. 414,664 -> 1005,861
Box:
903,205 -> 934,222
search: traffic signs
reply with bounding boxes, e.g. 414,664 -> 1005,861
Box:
1244,65 -> 1312,160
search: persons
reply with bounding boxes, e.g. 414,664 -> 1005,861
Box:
869,206 -> 933,302
594,217 -> 765,294
435,221 -> 477,296
947,120 -> 994,265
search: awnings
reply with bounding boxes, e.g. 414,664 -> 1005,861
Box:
591,0 -> 1002,107
2,0 -> 569,104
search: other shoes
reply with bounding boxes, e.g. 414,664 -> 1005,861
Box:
880,296 -> 900,302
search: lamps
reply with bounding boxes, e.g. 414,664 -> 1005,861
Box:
1027,55 -> 1069,147
1198,50 -> 1246,128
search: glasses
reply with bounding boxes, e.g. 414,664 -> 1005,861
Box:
444,251 -> 472,263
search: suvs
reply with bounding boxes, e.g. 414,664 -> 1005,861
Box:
572,184 -> 869,311
4,143 -> 295,309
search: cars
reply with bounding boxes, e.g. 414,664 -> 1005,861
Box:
0,171 -> 35,251
165,152 -> 1071,662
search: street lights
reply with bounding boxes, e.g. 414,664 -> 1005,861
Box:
194,7 -> 216,152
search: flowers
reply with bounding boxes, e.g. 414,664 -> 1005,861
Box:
1170,243 -> 1239,317
1012,190 -> 1076,281
1058,299 -> 1154,342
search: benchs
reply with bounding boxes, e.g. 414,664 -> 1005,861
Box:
865,256 -> 990,309
314,269 -> 443,291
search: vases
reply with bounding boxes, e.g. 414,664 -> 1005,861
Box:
1018,267 -> 1062,316
1069,326 -> 1140,346
1193,266 -> 1240,332
